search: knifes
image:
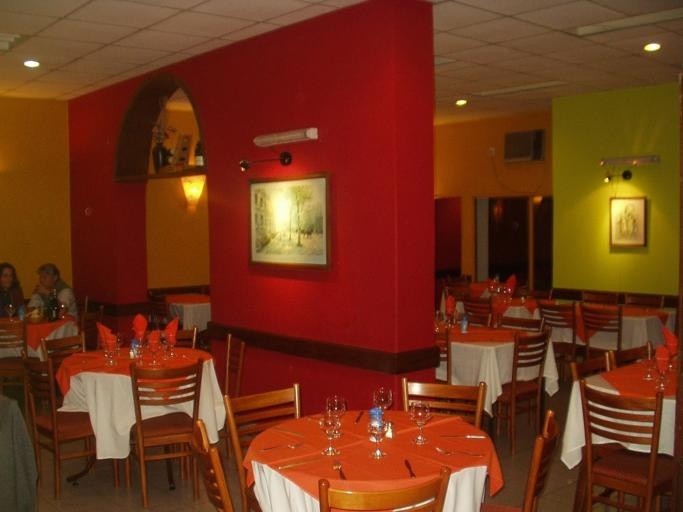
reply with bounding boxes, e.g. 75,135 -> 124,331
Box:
354,410 -> 363,425
403,458 -> 417,479
440,434 -> 489,439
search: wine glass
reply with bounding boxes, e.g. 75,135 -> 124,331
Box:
406,401 -> 431,447
367,418 -> 389,460
637,348 -> 679,392
316,414 -> 340,458
325,393 -> 345,439
5,303 -> 15,322
57,303 -> 67,319
374,385 -> 394,428
98,332 -> 174,368
433,279 -> 513,334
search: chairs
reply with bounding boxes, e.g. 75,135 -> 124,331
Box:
570,353 -> 611,377
609,341 -> 653,366
40,330 -> 85,360
430,277 -> 680,455
218,334 -> 246,397
1,319 -> 29,387
478,409 -> 559,512
20,358 -> 120,500
125,358 -> 205,512
223,383 -> 302,512
188,420 -> 233,512
78,295 -> 89,331
575,378 -> 672,511
147,327 -> 197,348
402,374 -> 485,431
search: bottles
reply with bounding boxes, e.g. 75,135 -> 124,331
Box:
48,288 -> 57,322
194,143 -> 204,166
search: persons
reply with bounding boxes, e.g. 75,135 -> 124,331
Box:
0,262 -> 25,320
31,262 -> 79,350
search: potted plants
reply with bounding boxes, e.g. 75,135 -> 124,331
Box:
195,143 -> 203,168
150,96 -> 178,171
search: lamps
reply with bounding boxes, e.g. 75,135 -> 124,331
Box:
179,175 -> 206,212
601,170 -> 633,184
238,151 -> 292,172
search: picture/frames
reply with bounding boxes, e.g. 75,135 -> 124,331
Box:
248,173 -> 329,278
607,195 -> 648,250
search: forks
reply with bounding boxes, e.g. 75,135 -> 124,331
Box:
435,447 -> 485,460
258,441 -> 304,451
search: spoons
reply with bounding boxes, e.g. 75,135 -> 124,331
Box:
329,459 -> 347,481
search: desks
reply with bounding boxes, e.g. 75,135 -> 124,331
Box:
57,345 -> 228,461
4,309 -> 79,360
